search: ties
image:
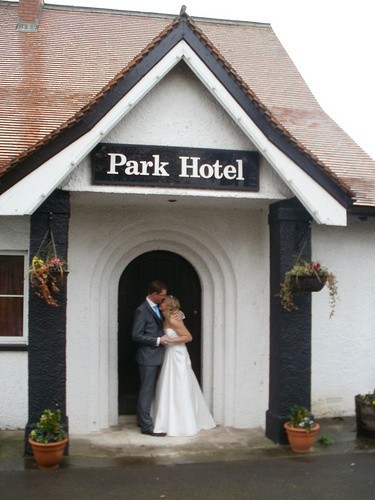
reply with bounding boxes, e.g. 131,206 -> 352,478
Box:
153,305 -> 160,319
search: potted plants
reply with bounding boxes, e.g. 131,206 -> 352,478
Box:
355,394 -> 375,437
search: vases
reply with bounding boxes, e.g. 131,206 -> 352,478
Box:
28,438 -> 67,469
294,276 -> 328,292
50,271 -> 69,279
284,422 -> 320,454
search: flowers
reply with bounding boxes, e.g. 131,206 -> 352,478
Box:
27,254 -> 67,306
290,405 -> 315,434
29,409 -> 66,444
274,255 -> 338,317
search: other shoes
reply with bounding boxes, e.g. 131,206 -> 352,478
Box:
141,425 -> 166,436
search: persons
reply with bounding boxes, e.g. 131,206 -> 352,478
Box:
133,281 -> 186,436
150,295 -> 216,437
